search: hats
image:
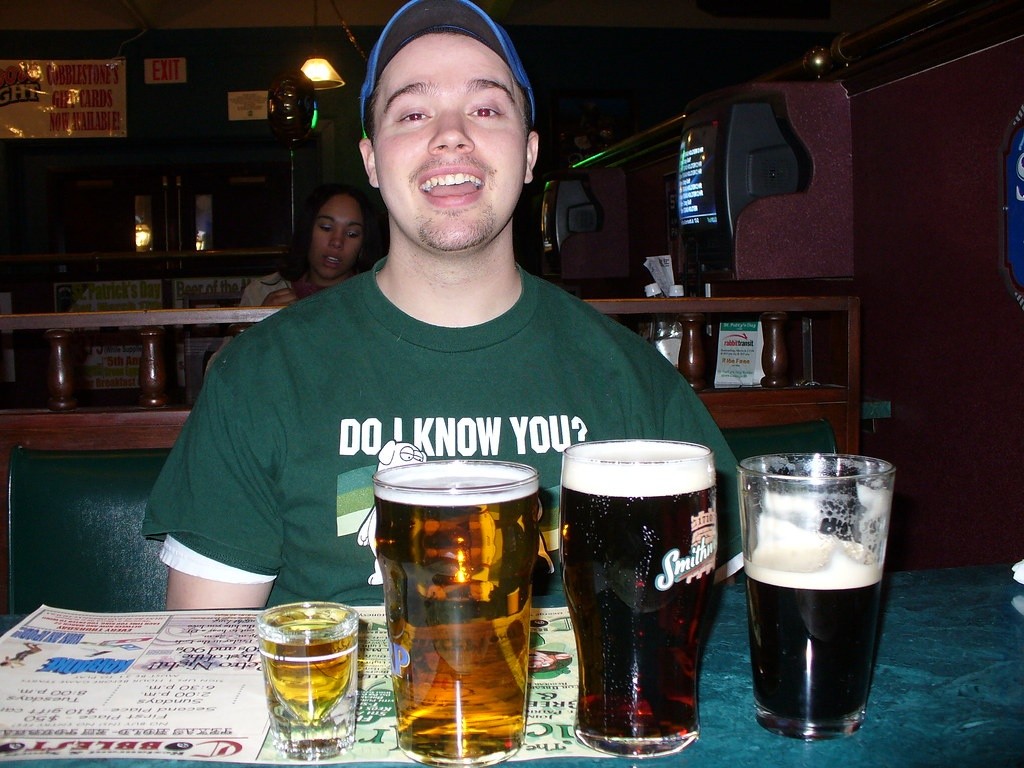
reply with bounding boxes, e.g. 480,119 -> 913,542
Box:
361,0 -> 535,138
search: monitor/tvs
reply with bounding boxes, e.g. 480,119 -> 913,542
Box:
669,93 -> 808,263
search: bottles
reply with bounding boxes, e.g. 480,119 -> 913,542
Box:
172,282 -> 190,308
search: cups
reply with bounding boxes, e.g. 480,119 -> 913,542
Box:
560,438 -> 718,757
372,460 -> 539,764
256,601 -> 360,762
738,452 -> 896,740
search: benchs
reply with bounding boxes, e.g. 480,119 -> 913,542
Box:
0,296 -> 862,616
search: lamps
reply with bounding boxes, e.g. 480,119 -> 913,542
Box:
300,0 -> 368,90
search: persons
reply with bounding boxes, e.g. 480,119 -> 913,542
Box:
142,1 -> 754,611
204,182 -> 381,383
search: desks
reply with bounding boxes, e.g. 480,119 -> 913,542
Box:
1,559 -> 1024,767
